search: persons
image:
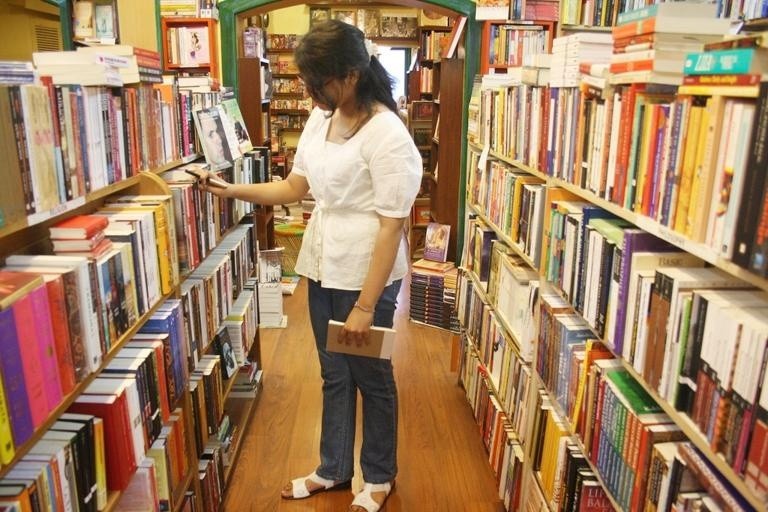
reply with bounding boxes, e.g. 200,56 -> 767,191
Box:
190,18 -> 426,512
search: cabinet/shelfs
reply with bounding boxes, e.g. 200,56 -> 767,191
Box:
1,0 -> 768,512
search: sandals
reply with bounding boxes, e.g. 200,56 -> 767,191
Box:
279,466 -> 354,500
348,474 -> 397,512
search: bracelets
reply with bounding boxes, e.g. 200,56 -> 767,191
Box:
352,301 -> 378,315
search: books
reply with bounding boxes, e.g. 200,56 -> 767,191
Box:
410,222 -> 463,334
324,319 -> 398,363
410,30 -> 451,225
243,30 -> 312,155
271,155 -> 314,224
0,39 -> 284,511
453,0 -> 768,512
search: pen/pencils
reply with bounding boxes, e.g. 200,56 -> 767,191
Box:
184,169 -> 227,189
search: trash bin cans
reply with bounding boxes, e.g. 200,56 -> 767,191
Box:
274,223 -> 306,276
